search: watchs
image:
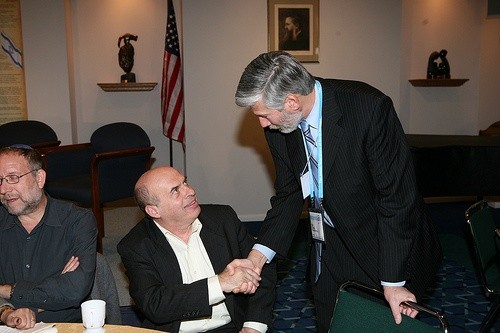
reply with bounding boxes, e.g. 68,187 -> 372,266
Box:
10,285 -> 16,300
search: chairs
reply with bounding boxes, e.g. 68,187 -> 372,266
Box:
40,122 -> 157,253
463,199 -> 500,333
326,279 -> 452,333
0,119 -> 61,153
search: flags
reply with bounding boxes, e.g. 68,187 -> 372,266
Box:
160,0 -> 186,150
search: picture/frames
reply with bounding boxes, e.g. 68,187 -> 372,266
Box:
266,0 -> 320,64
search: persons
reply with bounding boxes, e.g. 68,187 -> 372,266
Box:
225,51 -> 430,333
0,147 -> 98,333
0,299 -> 37,330
116,166 -> 276,333
280,11 -> 306,50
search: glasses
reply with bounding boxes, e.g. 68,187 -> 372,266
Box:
0,167 -> 41,186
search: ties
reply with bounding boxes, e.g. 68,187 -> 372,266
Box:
299,121 -> 323,285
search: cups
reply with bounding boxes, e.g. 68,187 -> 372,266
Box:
81,299 -> 106,328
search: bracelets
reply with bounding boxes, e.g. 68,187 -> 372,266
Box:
0,306 -> 18,319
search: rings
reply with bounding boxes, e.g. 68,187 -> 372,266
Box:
243,276 -> 247,280
